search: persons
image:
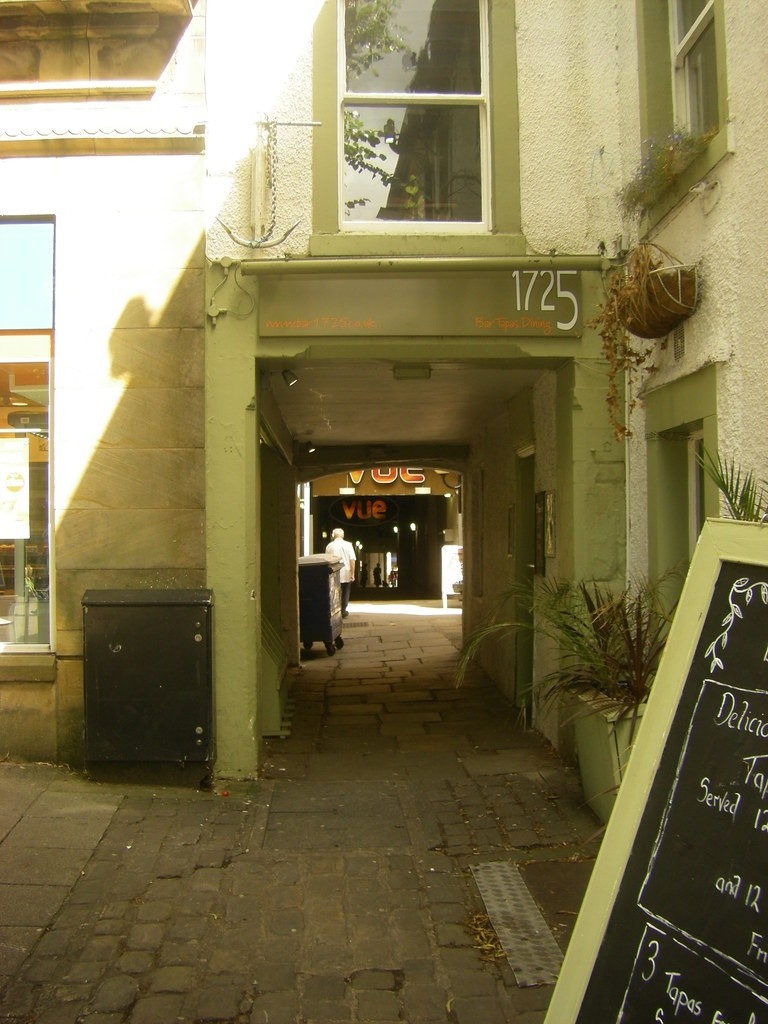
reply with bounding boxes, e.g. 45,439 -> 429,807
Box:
374,563 -> 382,589
361,562 -> 368,588
325,529 -> 357,617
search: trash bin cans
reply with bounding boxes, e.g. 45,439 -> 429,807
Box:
297,552 -> 344,656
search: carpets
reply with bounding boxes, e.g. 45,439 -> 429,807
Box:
517,858 -> 598,957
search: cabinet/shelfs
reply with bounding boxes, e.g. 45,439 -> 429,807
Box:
81,588 -> 218,791
0,544 -> 49,595
298,561 -> 344,655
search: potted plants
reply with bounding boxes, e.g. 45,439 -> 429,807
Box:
454,561 -> 689,826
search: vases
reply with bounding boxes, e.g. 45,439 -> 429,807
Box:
615,267 -> 699,338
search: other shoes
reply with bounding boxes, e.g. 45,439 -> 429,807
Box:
342,611 -> 349,619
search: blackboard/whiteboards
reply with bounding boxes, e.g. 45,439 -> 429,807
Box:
537,517 -> 768,1024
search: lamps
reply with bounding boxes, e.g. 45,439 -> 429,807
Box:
305,440 -> 316,454
282,369 -> 299,388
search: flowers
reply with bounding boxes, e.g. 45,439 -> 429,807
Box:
613,122 -> 711,229
